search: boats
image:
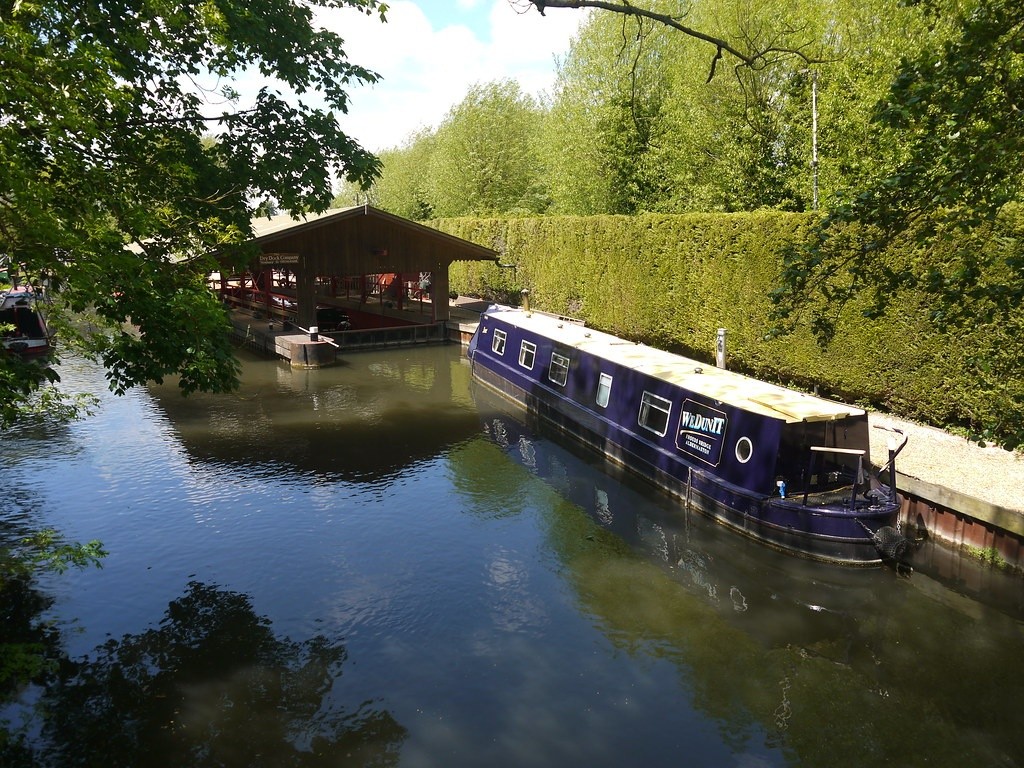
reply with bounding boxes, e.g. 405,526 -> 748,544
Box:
0,292 -> 52,356
467,288 -> 911,570
